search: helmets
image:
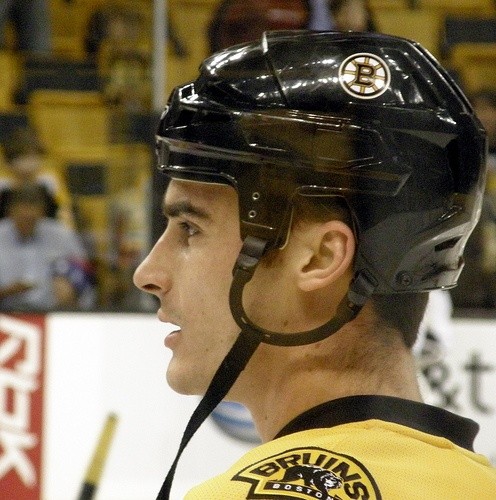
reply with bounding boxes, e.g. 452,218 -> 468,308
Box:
154,28 -> 488,348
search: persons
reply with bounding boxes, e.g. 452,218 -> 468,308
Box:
131,30 -> 496,500
0,0 -> 496,313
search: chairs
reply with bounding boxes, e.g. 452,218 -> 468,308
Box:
0,12 -> 496,270
